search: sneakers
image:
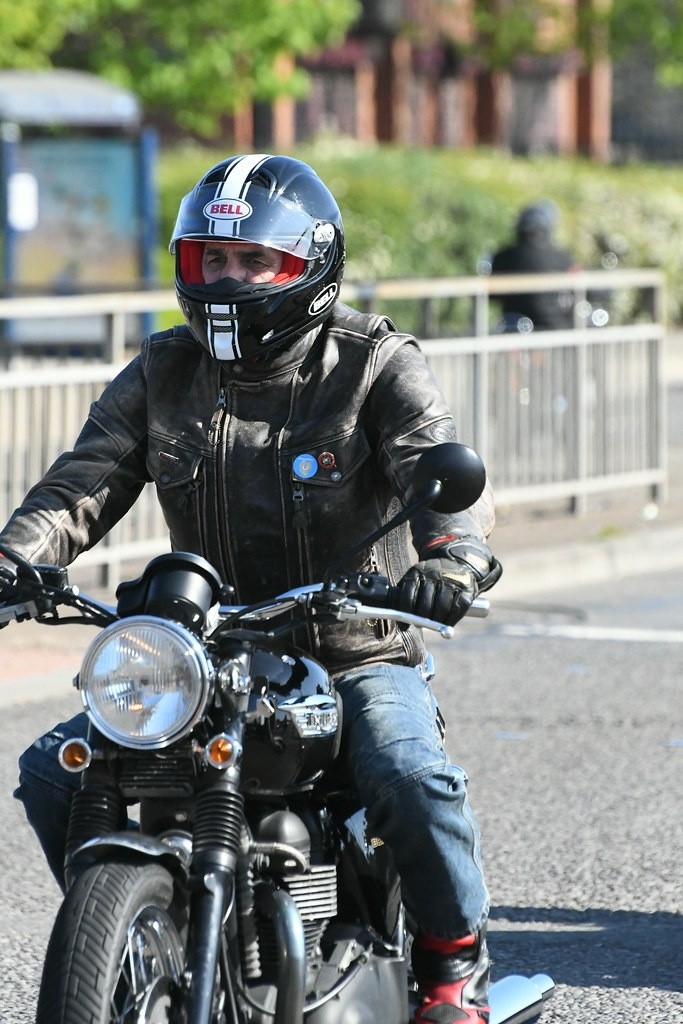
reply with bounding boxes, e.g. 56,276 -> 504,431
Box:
408,919 -> 490,1024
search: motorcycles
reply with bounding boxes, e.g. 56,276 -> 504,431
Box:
0,440 -> 558,1023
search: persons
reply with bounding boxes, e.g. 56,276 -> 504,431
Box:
0,153 -> 502,1024
492,199 -> 576,334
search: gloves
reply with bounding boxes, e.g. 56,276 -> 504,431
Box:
0,550 -> 19,630
394,538 -> 502,633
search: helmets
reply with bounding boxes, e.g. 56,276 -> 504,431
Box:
169,154 -> 346,361
517,201 -> 553,231
